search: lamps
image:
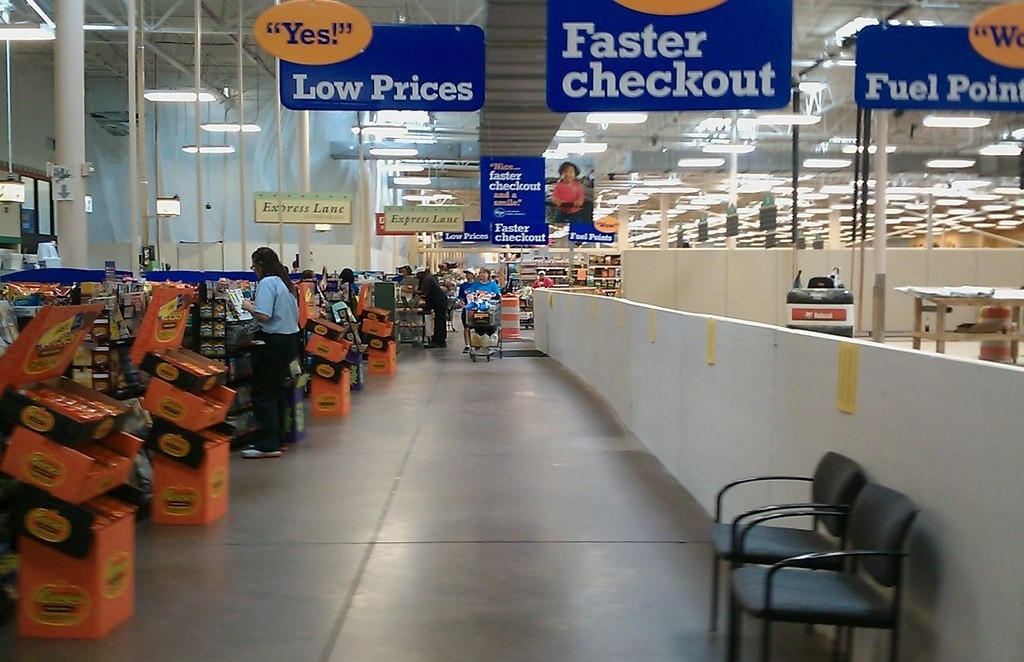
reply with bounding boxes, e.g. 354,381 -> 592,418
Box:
199,68 -> 264,132
148,194 -> 181,218
144,87 -> 233,101
181,102 -> 237,154
350,118 -> 452,202
0,25 -> 56,41
1,41 -> 25,214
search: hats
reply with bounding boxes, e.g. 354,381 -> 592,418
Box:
462,268 -> 475,276
538,270 -> 546,276
412,267 -> 426,274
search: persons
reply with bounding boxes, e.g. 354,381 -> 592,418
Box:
411,266 -> 452,348
457,267 -> 479,353
302,265 -> 327,288
554,161 -> 586,215
470,265 -> 501,294
399,265 -> 411,275
241,245 -> 300,458
532,270 -> 554,288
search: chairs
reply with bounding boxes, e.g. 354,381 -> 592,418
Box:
726,481 -> 926,662
710,451 -> 867,662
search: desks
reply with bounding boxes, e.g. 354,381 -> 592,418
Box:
913,286 -> 1024,363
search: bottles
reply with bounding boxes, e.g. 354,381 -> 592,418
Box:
71,282 -> 81,305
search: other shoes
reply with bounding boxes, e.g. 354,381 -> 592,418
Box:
281,444 -> 289,450
423,342 -> 446,347
463,346 -> 470,353
239,445 -> 283,459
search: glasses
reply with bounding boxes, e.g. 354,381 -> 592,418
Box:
250,265 -> 256,271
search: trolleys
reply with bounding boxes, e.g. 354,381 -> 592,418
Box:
468,304 -> 503,362
519,298 -> 534,328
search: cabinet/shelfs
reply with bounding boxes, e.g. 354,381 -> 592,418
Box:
0,286 -> 432,451
498,257 -> 624,330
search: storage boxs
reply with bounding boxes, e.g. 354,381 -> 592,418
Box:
129,286 -> 228,394
356,282 -> 394,349
299,281 -> 344,340
0,303 -> 134,447
